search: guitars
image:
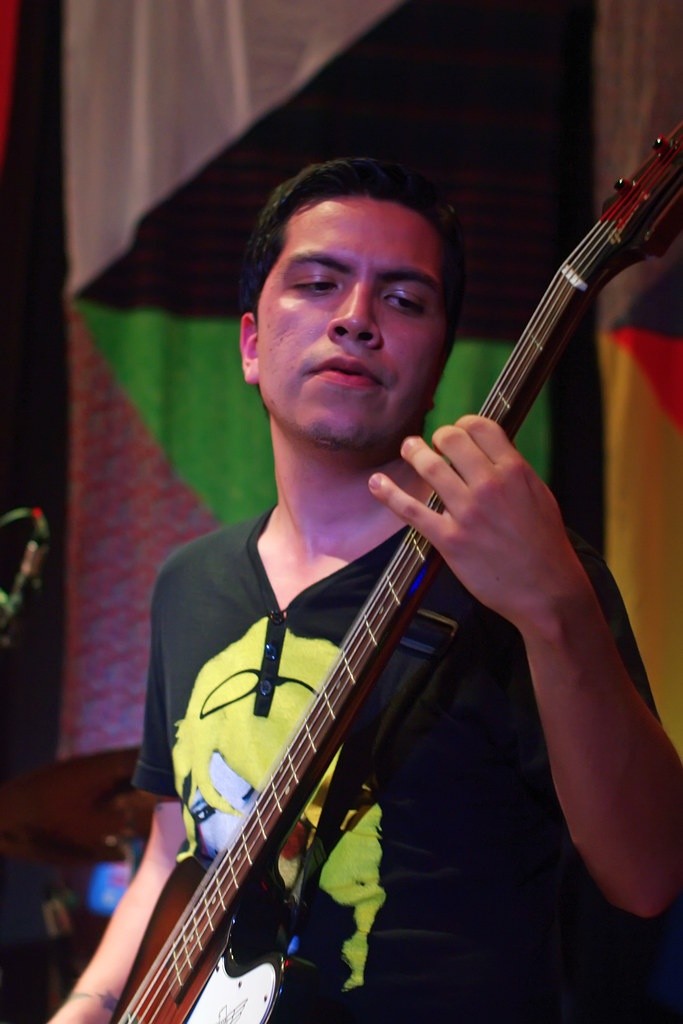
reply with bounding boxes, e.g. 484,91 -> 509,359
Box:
106,120 -> 683,1024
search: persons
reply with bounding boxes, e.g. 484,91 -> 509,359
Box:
45,158 -> 683,1024
